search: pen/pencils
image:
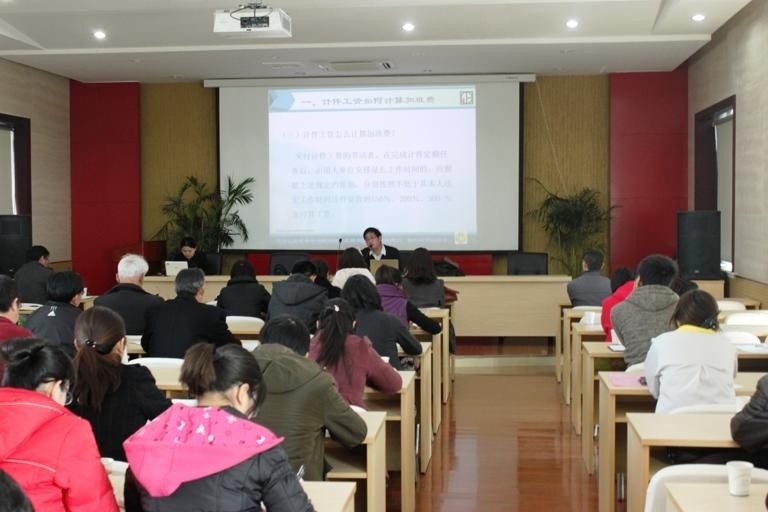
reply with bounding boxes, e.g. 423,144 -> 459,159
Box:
297,465 -> 304,476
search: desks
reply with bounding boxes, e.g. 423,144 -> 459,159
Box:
131,274 -> 572,338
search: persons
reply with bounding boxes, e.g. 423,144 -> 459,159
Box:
600,265 -> 643,343
360,227 -> 404,273
159,236 -> 218,276
642,287 -> 738,412
0,244 -> 457,483
122,340 -> 316,512
609,253 -> 682,371
728,373 -> 768,470
565,248 -> 613,307
0,337 -> 119,511
672,278 -> 699,298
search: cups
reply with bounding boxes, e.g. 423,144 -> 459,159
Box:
725,460 -> 753,497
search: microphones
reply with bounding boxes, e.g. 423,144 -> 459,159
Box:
337,238 -> 342,267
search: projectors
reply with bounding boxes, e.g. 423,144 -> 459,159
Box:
212,8 -> 292,40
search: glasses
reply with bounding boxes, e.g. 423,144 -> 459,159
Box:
248,395 -> 262,417
41,377 -> 74,406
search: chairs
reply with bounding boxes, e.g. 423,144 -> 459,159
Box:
504,249 -> 548,274
200,254 -> 223,273
268,249 -> 431,274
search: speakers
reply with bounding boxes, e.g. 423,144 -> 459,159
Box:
675,210 -> 721,280
0,215 -> 31,278
